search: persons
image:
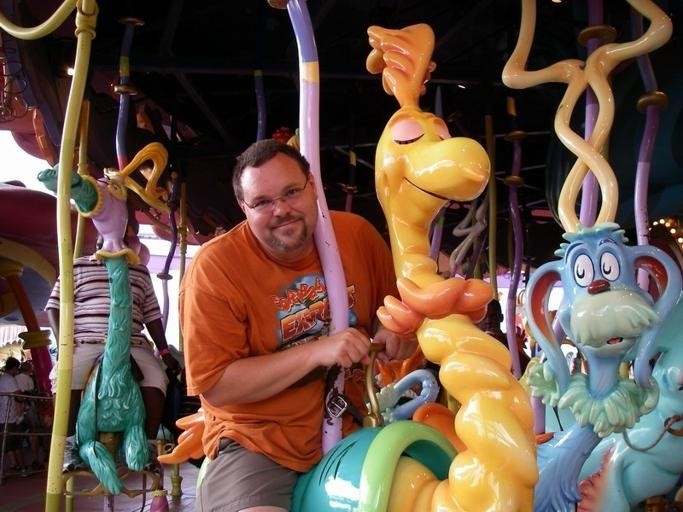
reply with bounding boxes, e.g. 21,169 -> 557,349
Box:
178,138 -> 425,512
0,357 -> 46,486
478,298 -> 531,376
42,234 -> 182,475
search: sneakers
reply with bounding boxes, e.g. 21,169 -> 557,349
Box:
9,461 -> 45,479
62,445 -> 88,472
143,442 -> 162,477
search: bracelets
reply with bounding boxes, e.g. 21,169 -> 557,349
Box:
159,347 -> 170,356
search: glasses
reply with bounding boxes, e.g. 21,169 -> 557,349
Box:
240,174 -> 309,214
